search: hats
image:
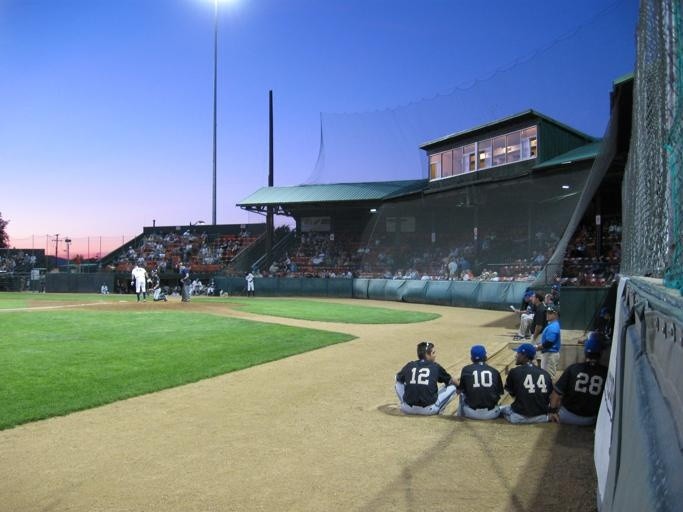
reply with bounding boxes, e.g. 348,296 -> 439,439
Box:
470,345 -> 486,362
510,343 -> 537,359
521,284 -> 561,302
546,305 -> 558,316
585,337 -> 606,361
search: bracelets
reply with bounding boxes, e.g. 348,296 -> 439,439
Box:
548,407 -> 558,413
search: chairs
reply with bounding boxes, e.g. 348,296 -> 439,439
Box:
260,227 -> 565,282
560,222 -> 618,282
114,232 -> 255,275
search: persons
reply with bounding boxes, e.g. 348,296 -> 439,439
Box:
395,342 -> 460,416
0,252 -> 46,293
499,343 -> 553,424
548,340 -> 608,427
457,345 -> 504,420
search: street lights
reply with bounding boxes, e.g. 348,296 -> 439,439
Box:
64,238 -> 70,271
